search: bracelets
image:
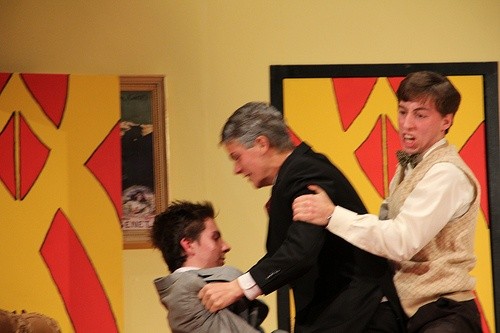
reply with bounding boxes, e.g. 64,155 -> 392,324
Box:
327,214 -> 331,225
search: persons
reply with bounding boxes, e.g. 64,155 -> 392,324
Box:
292,68 -> 483,333
149,200 -> 287,333
198,102 -> 403,333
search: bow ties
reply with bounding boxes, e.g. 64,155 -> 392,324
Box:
396,150 -> 422,169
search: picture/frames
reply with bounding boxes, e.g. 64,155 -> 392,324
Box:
266,62 -> 500,333
117,74 -> 169,251
